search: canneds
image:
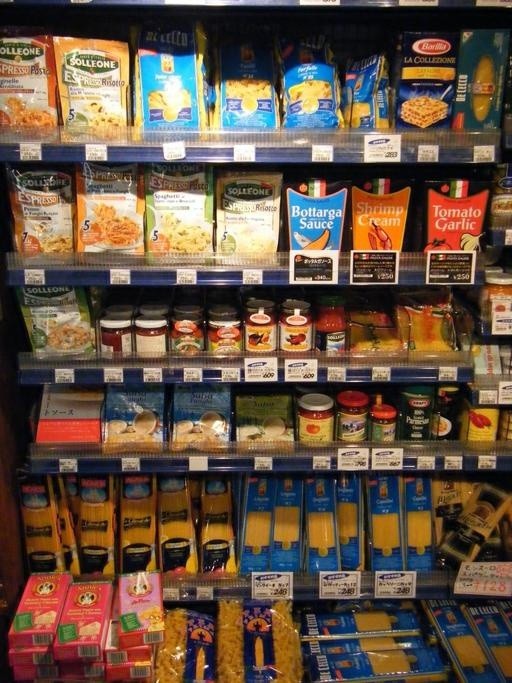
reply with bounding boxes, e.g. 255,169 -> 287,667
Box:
100,300 -> 314,355
296,390 -> 395,442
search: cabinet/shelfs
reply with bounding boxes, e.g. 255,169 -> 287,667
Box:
2,1 -> 512,602
